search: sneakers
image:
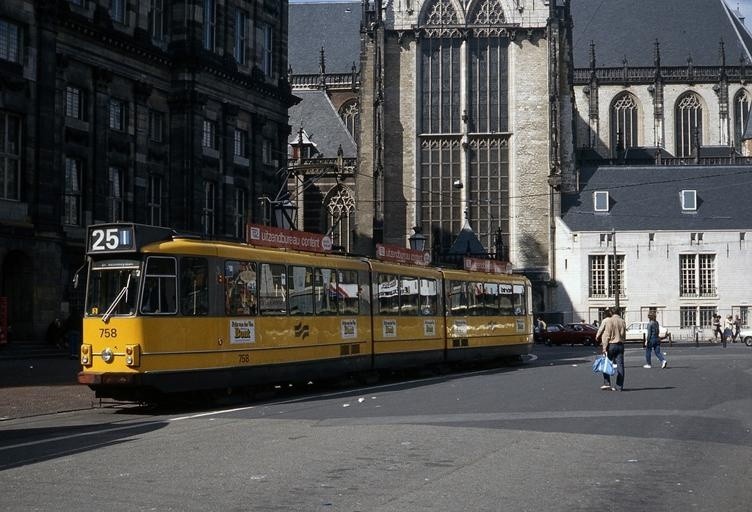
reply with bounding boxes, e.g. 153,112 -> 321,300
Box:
644,365 -> 651,368
662,360 -> 667,369
600,385 -> 616,391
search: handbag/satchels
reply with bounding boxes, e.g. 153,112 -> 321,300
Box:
593,357 -> 616,376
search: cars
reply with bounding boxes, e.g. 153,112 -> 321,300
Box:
739,325 -> 752,347
69,335 -> 78,360
535,322 -> 671,346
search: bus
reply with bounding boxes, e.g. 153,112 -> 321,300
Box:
72,222 -> 535,407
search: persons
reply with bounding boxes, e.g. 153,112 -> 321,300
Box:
537,317 -> 550,345
595,309 -> 617,368
643,311 -> 667,369
600,306 -> 626,392
708,314 -> 744,344
